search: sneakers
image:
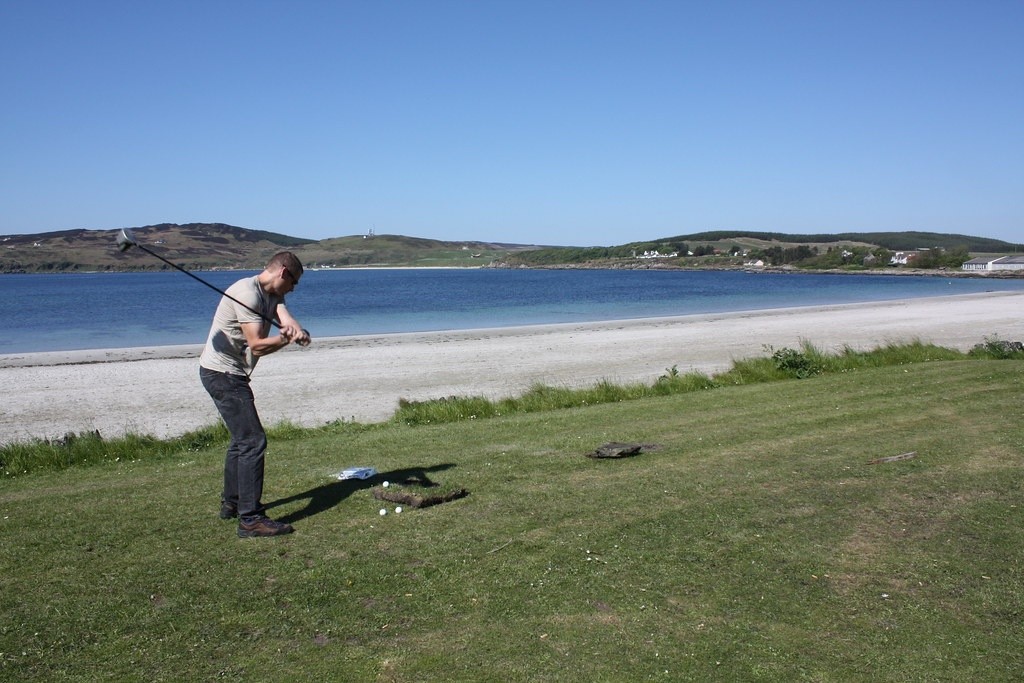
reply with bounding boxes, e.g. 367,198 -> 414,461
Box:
220,503 -> 263,519
238,517 -> 292,537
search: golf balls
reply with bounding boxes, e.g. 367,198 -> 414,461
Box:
395,507 -> 403,514
379,508 -> 386,515
383,481 -> 390,488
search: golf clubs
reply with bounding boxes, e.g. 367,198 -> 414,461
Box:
114,227 -> 284,332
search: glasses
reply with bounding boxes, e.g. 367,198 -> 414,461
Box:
282,264 -> 298,285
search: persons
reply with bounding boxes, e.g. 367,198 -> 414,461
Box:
199,252 -> 311,538
